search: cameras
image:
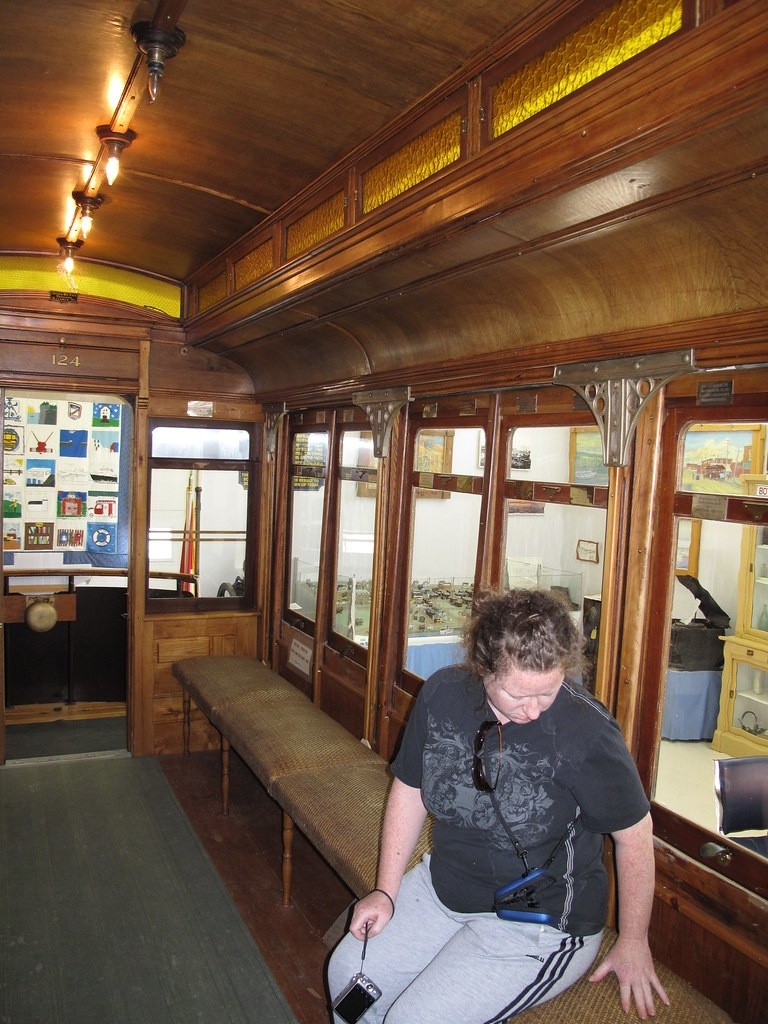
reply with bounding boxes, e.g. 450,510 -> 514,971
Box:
332,973 -> 382,1024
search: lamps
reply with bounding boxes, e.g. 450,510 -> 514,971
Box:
128,21 -> 187,107
54,237 -> 84,276
75,196 -> 106,240
95,124 -> 139,187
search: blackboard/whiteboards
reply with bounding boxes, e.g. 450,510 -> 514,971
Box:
238,429 -> 328,492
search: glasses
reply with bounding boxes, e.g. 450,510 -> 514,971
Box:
471,720 -> 502,793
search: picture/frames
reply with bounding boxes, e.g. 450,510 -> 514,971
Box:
676,519 -> 702,577
569,425 -> 766,501
358,430 -> 452,500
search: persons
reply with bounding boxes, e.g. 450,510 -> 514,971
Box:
327,587 -> 671,1024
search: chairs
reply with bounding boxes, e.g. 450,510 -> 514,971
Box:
716,756 -> 768,860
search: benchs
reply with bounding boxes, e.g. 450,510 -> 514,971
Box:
174,650 -> 735,1023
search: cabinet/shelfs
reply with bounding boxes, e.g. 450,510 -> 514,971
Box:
711,470 -> 767,757
